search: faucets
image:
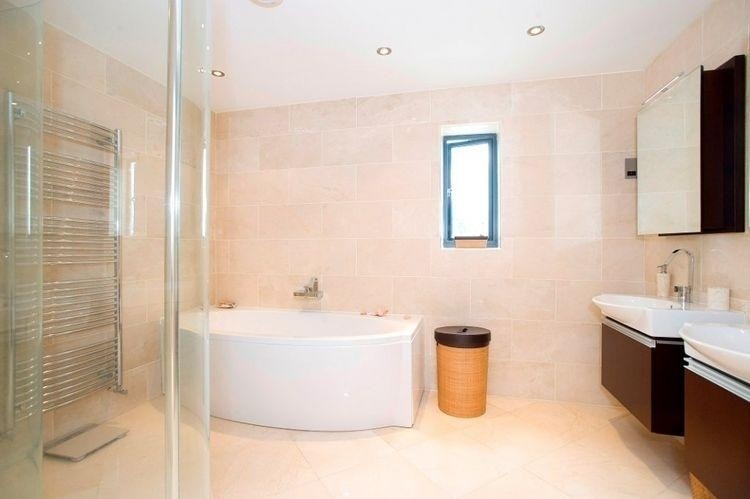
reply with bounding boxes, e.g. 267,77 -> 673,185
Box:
665,249 -> 695,303
304,278 -> 324,302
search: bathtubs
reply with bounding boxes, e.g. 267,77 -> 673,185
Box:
159,305 -> 424,432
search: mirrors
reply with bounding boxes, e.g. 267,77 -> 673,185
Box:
636,66 -> 701,235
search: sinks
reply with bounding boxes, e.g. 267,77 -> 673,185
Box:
680,322 -> 750,385
593,293 -> 746,338
